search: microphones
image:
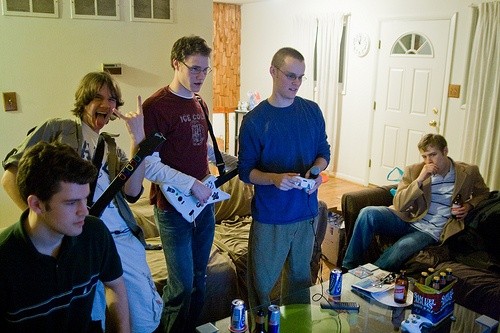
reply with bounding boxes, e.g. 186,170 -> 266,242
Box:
308,167 -> 320,180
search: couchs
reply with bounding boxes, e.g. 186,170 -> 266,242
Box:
105,175 -> 328,333
337,184 -> 500,315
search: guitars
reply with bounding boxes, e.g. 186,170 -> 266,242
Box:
159,166 -> 241,223
89,131 -> 167,219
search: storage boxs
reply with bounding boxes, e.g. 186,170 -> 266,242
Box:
413,272 -> 457,324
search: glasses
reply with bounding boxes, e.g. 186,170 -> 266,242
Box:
274,65 -> 307,82
181,60 -> 212,76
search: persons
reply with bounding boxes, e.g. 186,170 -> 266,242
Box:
237,47 -> 331,304
1,72 -> 165,333
342,134 -> 490,274
144,36 -> 239,333
0,140 -> 131,333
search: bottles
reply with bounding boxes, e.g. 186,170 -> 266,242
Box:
451,194 -> 463,219
253,310 -> 267,333
394,270 -> 409,304
419,268 -> 453,290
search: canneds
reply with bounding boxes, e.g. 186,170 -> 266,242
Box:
329,269 -> 343,296
231,299 -> 245,330
267,305 -> 280,333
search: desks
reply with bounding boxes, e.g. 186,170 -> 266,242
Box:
198,263 -> 498,333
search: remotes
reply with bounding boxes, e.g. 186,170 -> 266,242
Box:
320,302 -> 360,310
